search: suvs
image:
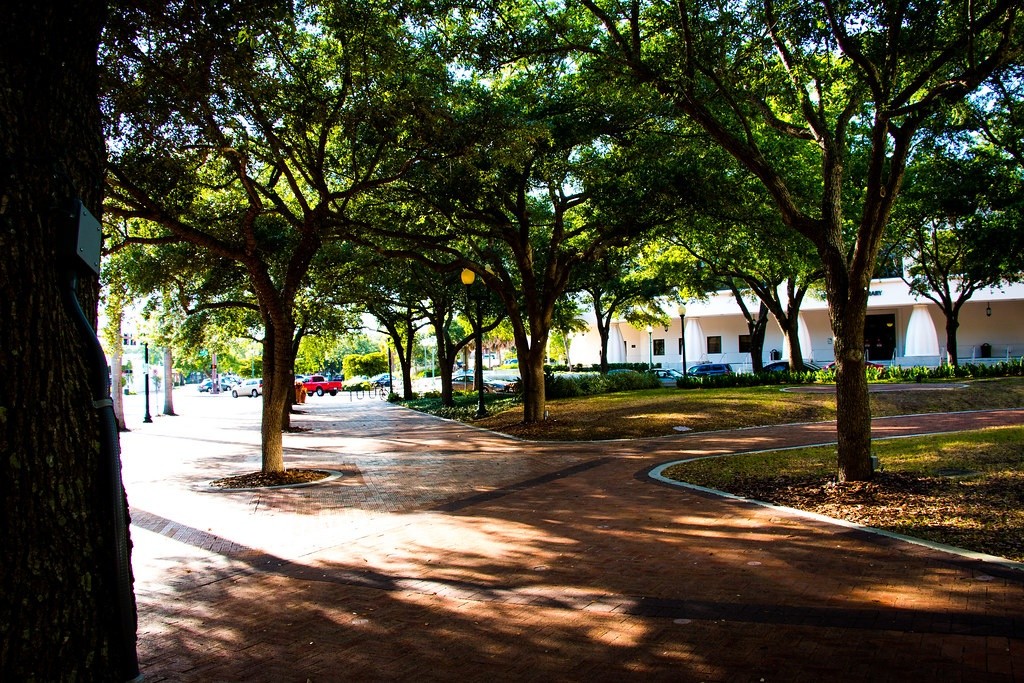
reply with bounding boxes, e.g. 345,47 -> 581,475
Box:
686,362 -> 734,377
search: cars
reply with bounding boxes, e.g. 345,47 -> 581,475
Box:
198,375 -> 243,393
295,373 -> 306,386
333,372 -> 398,392
765,359 -> 821,374
452,373 -> 508,392
652,369 -> 684,387
231,377 -> 263,399
821,361 -> 884,372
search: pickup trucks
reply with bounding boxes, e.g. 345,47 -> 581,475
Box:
301,375 -> 343,397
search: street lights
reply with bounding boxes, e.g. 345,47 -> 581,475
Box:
462,265 -> 494,418
677,302 -> 687,376
646,324 -> 653,370
